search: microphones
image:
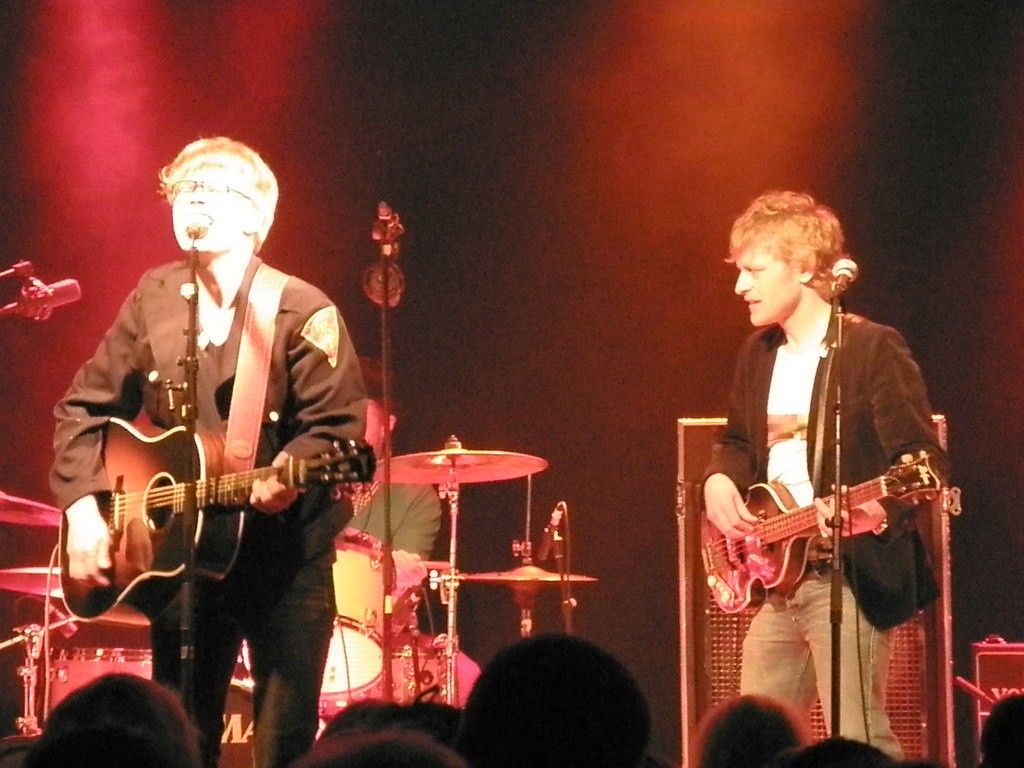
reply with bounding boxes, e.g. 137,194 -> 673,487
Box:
377,201 -> 400,242
831,258 -> 858,294
42,601 -> 77,638
185,220 -> 207,238
0,279 -> 81,321
389,588 -> 427,636
537,502 -> 564,561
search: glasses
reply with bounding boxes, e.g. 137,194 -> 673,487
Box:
172,175 -> 273,224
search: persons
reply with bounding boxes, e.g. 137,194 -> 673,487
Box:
325,352 -> 483,707
695,188 -> 954,762
48,134 -> 368,768
1,632 -> 1023,768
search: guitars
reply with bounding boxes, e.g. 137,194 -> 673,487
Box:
58,417 -> 377,626
700,450 -> 941,614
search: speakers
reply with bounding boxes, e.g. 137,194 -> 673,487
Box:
675,417 -> 952,768
971,641 -> 1024,761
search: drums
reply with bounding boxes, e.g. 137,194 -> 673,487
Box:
49,647 -> 153,714
241,525 -> 397,699
217,680 -> 254,768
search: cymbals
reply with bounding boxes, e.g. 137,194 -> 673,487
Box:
0,566 -> 63,599
372,448 -> 549,484
457,565 -> 600,593
0,491 -> 59,528
